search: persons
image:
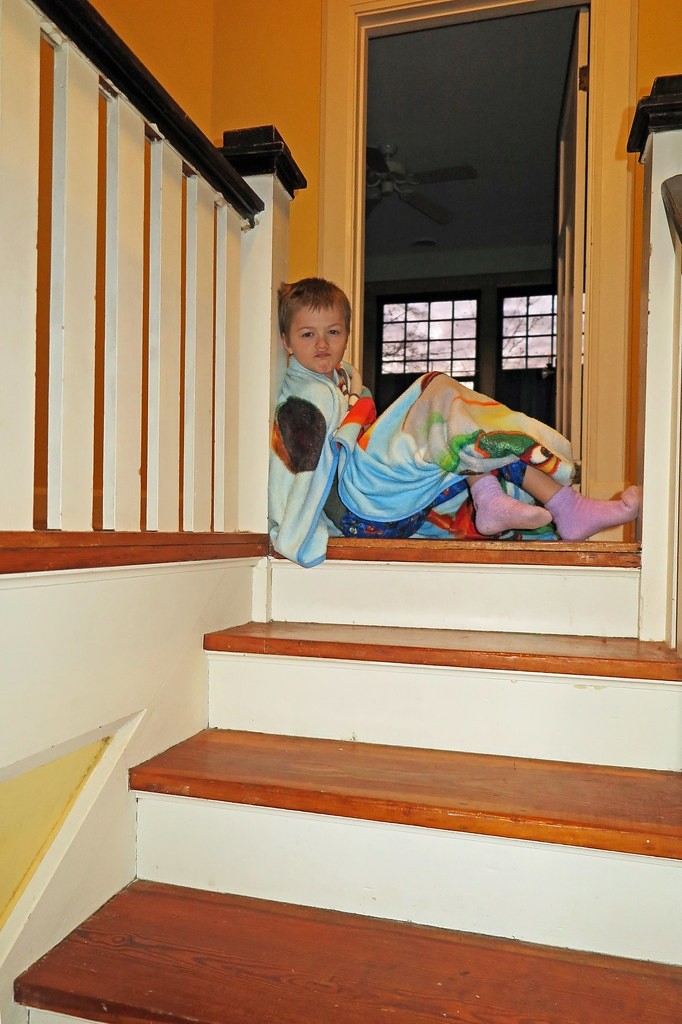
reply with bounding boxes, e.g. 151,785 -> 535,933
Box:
275,278 -> 641,543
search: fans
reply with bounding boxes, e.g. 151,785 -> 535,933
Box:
366,141 -> 479,226
